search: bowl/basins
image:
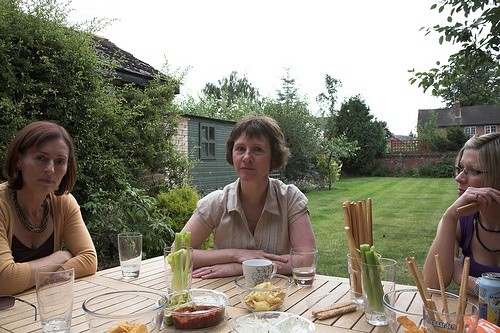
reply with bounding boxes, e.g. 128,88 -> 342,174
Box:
157,289 -> 229,330
382,288 -> 459,333
234,273 -> 291,312
81,290 -> 167,333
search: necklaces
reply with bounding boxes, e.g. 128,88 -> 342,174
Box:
12,188 -> 49,233
474,211 -> 500,252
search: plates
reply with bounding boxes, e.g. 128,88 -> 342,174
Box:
462,314 -> 500,333
230,312 -> 316,333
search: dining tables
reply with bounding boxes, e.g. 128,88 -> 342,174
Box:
0,248 -> 464,333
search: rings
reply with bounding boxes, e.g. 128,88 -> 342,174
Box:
210,269 -> 212,273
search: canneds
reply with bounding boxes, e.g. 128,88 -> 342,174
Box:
478,272 -> 500,329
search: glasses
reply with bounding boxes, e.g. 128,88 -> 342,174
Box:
454,165 -> 493,177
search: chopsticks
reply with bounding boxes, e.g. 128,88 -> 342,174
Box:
405,254 -> 471,333
342,198 -> 373,297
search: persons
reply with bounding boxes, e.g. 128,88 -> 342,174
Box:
423,132 -> 500,292
170,115 -> 316,279
0,121 -> 97,296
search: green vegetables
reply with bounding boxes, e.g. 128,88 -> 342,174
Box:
163,231 -> 191,308
359,244 -> 389,312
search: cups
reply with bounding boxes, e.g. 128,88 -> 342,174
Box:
361,258 -> 397,326
290,247 -> 318,287
164,247 -> 193,296
118,232 -> 143,279
347,253 -> 381,304
35,264 -> 75,333
422,297 -> 480,333
241,259 -> 278,288
